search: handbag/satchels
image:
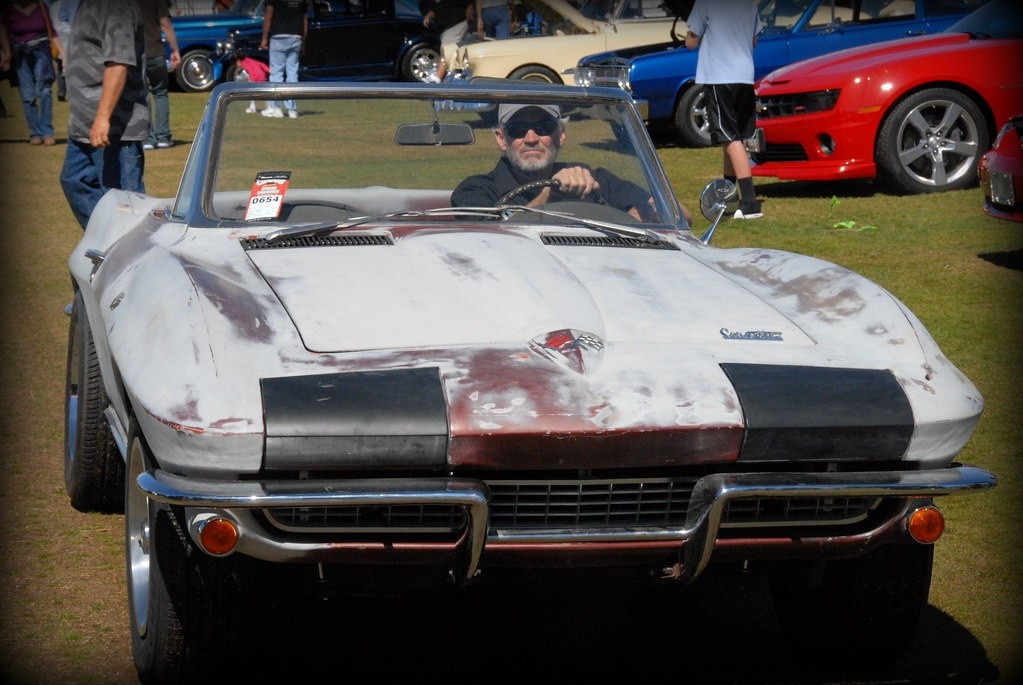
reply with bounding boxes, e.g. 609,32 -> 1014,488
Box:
50,41 -> 59,60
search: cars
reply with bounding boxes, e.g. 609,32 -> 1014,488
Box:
574,0 -> 1023,196
162,1 -> 441,92
65,80 -> 997,684
978,111 -> 1023,223
429,1 -> 874,127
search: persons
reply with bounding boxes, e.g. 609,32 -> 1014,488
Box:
0,0 -> 80,146
212,0 -> 236,14
451,102 -> 693,230
231,52 -> 270,117
59,0 -> 152,317
423,0 -> 475,83
476,0 -> 518,42
685,0 -> 765,220
137,0 -> 182,151
261,0 -> 309,118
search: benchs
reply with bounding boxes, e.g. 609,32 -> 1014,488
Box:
159,185 -> 453,221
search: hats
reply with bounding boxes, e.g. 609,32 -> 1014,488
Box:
498,103 -> 562,129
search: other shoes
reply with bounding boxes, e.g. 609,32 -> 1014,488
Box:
57,93 -> 65,101
246,105 -> 256,113
158,141 -> 173,148
143,144 -> 154,150
422,73 -> 441,84
729,209 -> 764,221
42,136 -> 55,145
30,136 -> 42,145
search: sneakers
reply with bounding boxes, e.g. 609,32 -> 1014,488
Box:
288,109 -> 301,119
261,106 -> 284,118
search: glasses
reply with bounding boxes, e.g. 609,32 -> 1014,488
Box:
505,118 -> 556,138
